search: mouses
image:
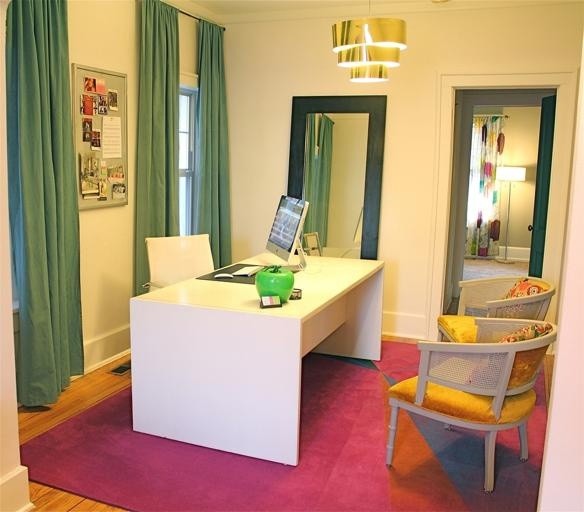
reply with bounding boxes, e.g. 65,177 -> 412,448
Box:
214,273 -> 234,279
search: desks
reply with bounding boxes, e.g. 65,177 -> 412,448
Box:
129,251 -> 385,467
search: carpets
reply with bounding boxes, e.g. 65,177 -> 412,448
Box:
18,339 -> 555,512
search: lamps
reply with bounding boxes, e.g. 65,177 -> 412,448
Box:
332,0 -> 406,82
495,165 -> 526,264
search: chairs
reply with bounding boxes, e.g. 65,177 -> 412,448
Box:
385,318 -> 557,493
302,232 -> 322,255
144,234 -> 216,292
435,275 -> 557,413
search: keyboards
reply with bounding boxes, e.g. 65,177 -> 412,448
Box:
232,265 -> 265,278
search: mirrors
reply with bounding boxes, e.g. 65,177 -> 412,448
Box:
286,94 -> 388,260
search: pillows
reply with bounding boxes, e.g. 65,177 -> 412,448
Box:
463,322 -> 552,395
505,278 -> 547,299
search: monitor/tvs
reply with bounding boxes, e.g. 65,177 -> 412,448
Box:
264,195 -> 309,273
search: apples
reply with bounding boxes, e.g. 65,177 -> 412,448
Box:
255,265 -> 294,303
